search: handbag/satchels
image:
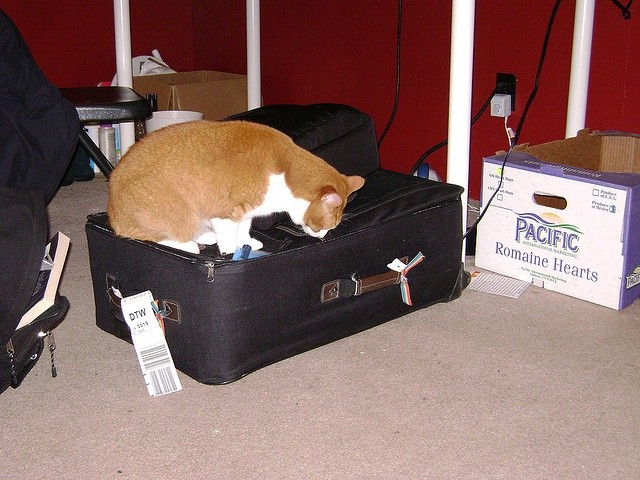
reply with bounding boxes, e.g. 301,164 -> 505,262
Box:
218,103 -> 379,232
0,191 -> 70,393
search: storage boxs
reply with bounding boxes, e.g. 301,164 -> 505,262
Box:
472,130 -> 640,314
130,68 -> 246,121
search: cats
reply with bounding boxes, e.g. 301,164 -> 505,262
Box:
107,117 -> 368,260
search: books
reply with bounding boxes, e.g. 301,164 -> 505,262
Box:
16,231 -> 70,330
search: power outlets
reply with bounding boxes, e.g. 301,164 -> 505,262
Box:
490,74 -> 518,118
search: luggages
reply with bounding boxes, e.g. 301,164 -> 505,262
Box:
85,168 -> 471,385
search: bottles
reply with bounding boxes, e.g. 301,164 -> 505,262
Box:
99,123 -> 117,167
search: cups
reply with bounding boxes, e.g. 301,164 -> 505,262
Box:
146,111 -> 204,134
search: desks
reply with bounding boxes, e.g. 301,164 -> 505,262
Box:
57,83 -> 153,174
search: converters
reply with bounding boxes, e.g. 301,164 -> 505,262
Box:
490,93 -> 512,117
